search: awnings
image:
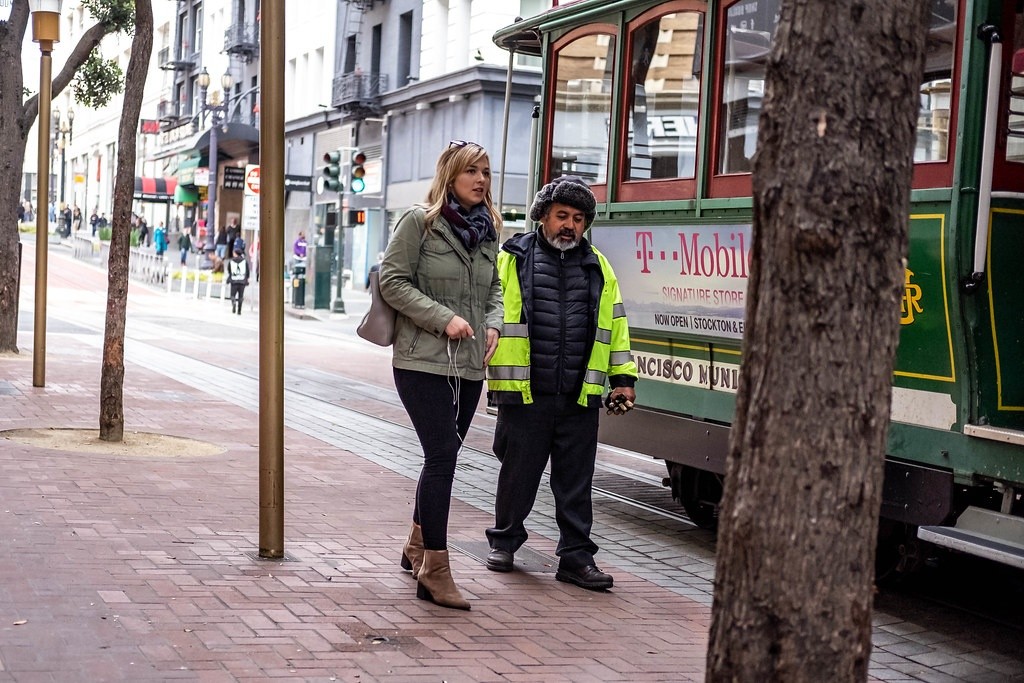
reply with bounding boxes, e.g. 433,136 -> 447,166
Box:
133,176 -> 177,204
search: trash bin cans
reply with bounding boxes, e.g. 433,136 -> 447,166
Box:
293,266 -> 306,307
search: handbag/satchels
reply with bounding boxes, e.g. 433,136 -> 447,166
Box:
357,264 -> 397,347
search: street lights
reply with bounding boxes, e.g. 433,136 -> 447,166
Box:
197,66 -> 234,270
51,105 -> 75,234
30,0 -> 62,389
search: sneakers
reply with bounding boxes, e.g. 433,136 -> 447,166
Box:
486,549 -> 514,572
556,565 -> 613,589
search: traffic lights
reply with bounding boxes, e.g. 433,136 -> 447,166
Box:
325,212 -> 337,227
350,209 -> 365,225
323,149 -> 344,192
350,150 -> 366,194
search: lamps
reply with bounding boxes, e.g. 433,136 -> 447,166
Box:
533,95 -> 541,103
448,95 -> 469,102
387,109 -> 407,116
416,102 -> 435,111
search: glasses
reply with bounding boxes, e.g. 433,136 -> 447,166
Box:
449,140 -> 484,149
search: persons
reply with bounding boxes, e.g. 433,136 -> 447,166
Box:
18,201 -> 32,222
178,228 -> 190,265
227,219 -> 246,257
226,248 -> 249,315
214,226 -> 227,258
64,204 -> 81,235
208,252 -> 224,273
379,144 -> 505,611
486,176 -> 639,589
294,231 -> 307,257
249,231 -> 260,282
153,221 -> 168,261
90,211 -> 148,247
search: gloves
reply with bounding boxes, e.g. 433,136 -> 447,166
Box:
605,390 -> 634,414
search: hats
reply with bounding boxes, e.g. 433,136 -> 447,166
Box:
529,175 -> 596,234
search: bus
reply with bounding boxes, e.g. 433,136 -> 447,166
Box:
487,2 -> 1024,571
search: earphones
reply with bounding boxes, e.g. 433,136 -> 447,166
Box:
471,335 -> 476,340
454,401 -> 456,405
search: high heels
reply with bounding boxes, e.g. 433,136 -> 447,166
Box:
401,523 -> 424,580
417,550 -> 471,610
